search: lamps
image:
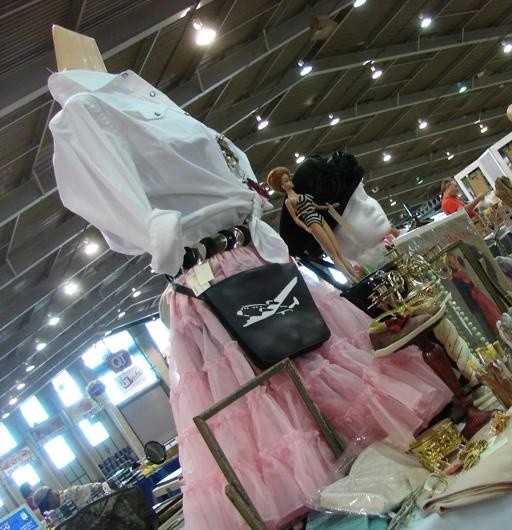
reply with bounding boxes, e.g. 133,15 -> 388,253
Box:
294,152 -> 305,164
296,57 -> 313,77
328,114 -> 340,125
192,18 -> 216,45
362,59 -> 382,80
255,114 -> 269,130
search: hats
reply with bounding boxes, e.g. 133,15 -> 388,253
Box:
32,485 -> 51,514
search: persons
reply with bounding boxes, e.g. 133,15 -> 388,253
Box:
441,179 -> 486,220
20,481 -> 34,511
447,253 -> 501,336
279,152 -> 392,265
32,483 -> 108,511
48,25 -> 454,530
267,167 -> 361,284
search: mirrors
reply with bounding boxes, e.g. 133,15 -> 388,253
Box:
495,139 -> 512,164
145,441 -> 165,464
460,167 -> 493,200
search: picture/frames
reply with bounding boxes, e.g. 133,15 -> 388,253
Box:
427,238 -> 510,368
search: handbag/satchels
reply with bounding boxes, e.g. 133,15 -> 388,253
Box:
203,263 -> 332,368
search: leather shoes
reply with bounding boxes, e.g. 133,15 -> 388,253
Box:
368,301 -> 441,351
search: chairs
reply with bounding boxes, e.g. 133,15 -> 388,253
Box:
55,486 -> 158,530
